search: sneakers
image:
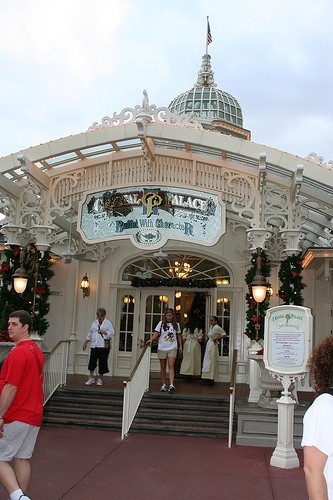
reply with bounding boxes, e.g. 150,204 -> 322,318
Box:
168,385 -> 176,392
160,384 -> 167,391
84,376 -> 95,385
96,378 -> 103,385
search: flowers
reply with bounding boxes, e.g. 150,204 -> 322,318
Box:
244,248 -> 271,354
278,251 -> 306,306
0,245 -> 53,342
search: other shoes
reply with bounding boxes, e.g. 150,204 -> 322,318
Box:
185,377 -> 193,381
200,379 -> 214,386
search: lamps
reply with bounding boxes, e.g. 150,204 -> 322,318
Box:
11,241 -> 38,330
80,273 -> 90,298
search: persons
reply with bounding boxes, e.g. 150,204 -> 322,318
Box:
146,308 -> 182,392
180,314 -> 203,375
83,308 -> 116,385
301,336 -> 333,500
202,315 -> 227,379
0,310 -> 44,500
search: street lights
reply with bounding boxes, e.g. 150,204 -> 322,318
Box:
250,246 -> 270,342
11,242 -> 38,334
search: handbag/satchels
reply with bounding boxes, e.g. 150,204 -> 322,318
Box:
105,339 -> 111,350
